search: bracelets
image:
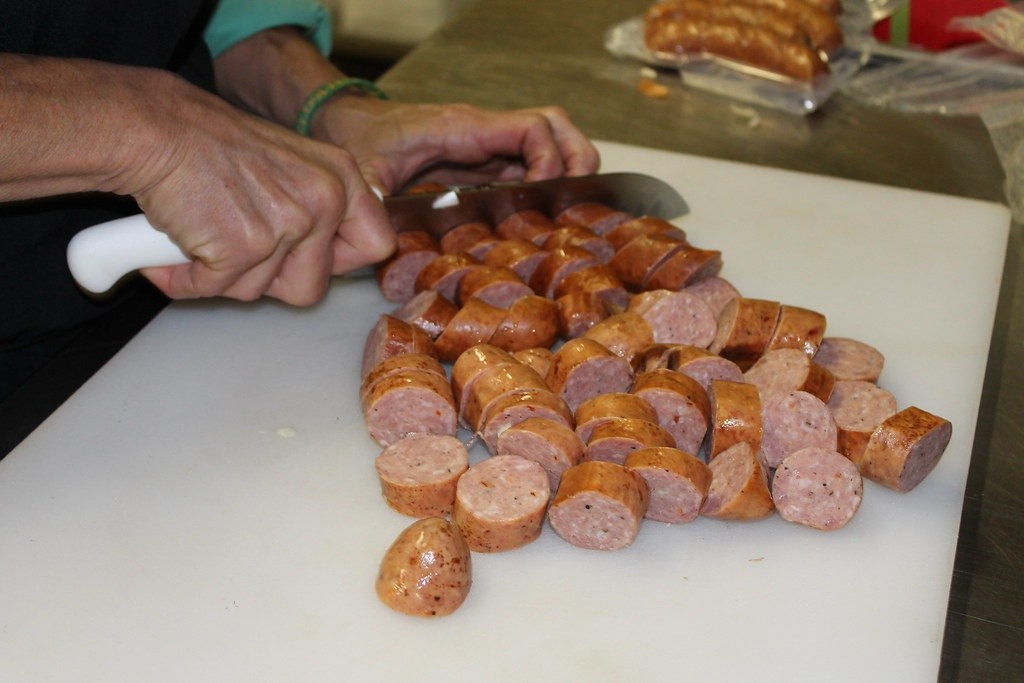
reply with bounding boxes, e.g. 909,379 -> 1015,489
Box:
296,78 -> 387,134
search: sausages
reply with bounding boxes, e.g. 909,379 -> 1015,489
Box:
644,0 -> 846,81
361,180 -> 952,618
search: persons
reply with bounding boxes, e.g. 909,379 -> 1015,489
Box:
0,0 -> 600,461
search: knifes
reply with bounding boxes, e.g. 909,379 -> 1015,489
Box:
66,172 -> 691,295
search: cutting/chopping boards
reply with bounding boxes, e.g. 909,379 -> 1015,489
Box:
1,136 -> 1012,683
